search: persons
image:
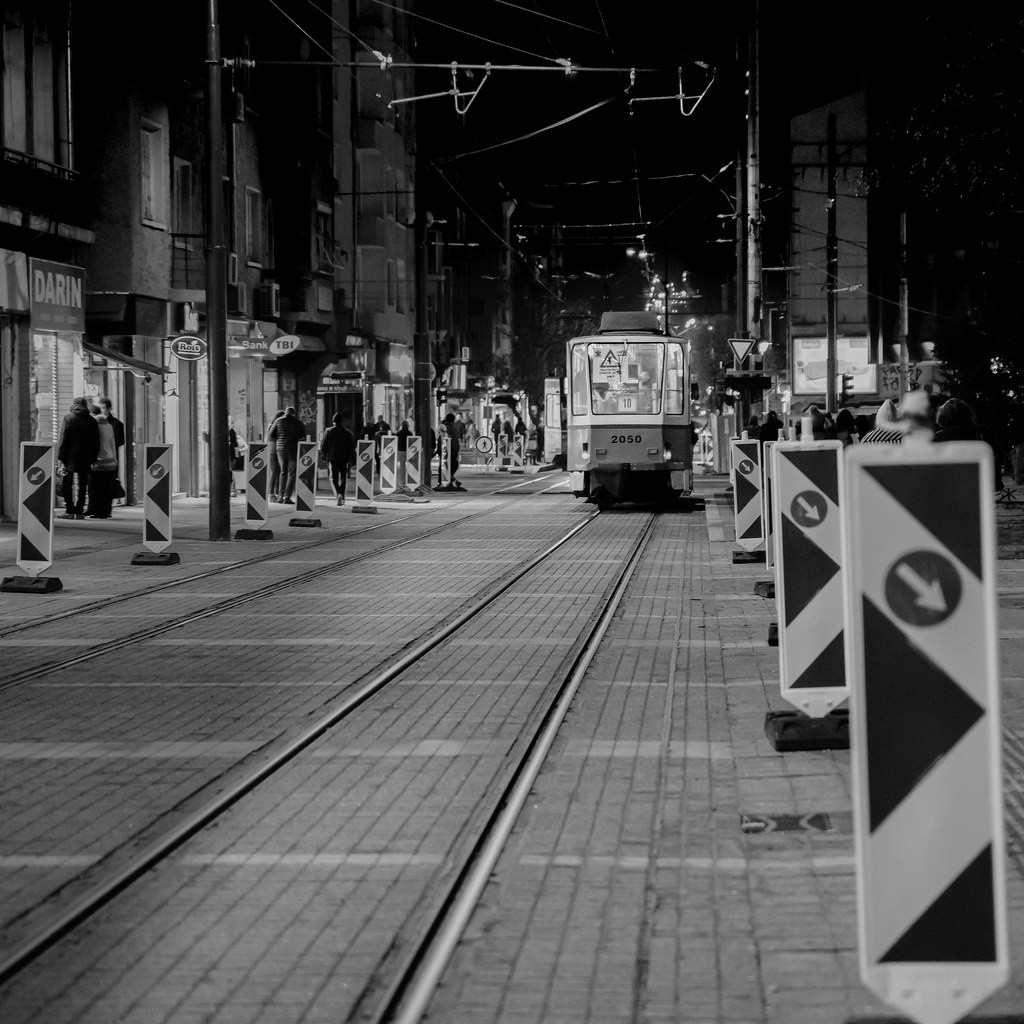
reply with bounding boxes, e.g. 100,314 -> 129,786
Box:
738,384 -> 1024,501
59,395 -> 101,520
88,404 -> 117,519
97,397 -> 126,513
225,401 -> 549,508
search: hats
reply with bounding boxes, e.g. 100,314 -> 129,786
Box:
332,412 -> 344,423
875,398 -> 899,430
768,411 -> 776,415
808,405 -> 825,428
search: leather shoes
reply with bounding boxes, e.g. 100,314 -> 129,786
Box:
56,513 -> 74,519
77,514 -> 84,519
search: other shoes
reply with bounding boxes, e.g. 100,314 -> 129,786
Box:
337,494 -> 343,506
270,495 -> 295,504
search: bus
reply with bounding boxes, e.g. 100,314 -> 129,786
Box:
562,309 -> 701,503
